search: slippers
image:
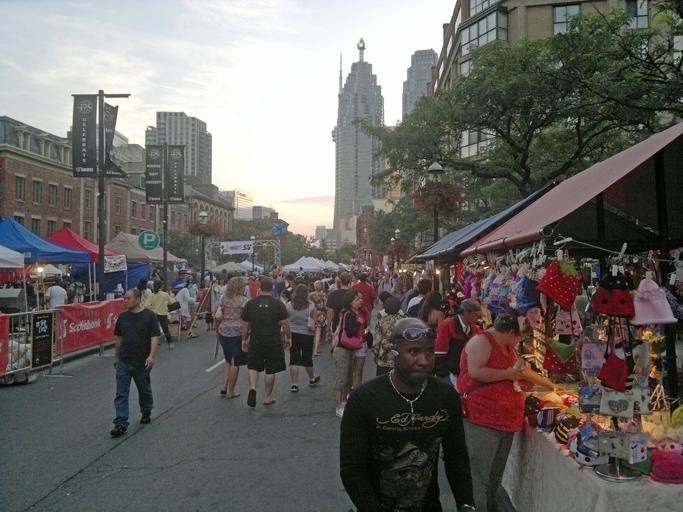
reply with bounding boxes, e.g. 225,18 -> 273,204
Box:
263,397 -> 276,405
246,389 -> 257,408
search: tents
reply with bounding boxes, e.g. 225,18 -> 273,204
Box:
208,261 -> 269,274
284,257 -> 339,272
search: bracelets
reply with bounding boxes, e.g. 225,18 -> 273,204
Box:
457,503 -> 475,511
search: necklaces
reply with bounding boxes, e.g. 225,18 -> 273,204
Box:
387,369 -> 427,425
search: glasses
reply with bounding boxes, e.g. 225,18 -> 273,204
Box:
391,326 -> 438,342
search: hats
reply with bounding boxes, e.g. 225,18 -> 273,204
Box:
457,296 -> 487,318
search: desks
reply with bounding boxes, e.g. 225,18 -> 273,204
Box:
496,397 -> 683,512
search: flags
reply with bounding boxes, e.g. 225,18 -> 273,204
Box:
72,95 -> 98,178
144,146 -> 163,203
100,101 -> 127,178
165,144 -> 184,203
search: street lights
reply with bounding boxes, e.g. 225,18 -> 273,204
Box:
249,234 -> 254,271
427,159 -> 445,242
197,210 -> 208,289
394,227 -> 401,265
389,237 -> 395,272
262,243 -> 266,274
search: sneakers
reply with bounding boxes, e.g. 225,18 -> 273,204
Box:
219,388 -> 240,399
335,401 -> 348,420
290,383 -> 299,393
110,422 -> 128,436
308,376 -> 321,387
139,411 -> 152,424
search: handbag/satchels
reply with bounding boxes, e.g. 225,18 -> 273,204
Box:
339,326 -> 364,352
213,304 -> 225,324
305,313 -> 317,332
166,300 -> 182,313
525,254 -> 683,485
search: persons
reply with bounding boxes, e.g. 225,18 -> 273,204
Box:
139,269 -> 292,407
286,266 -> 446,393
109,287 -> 162,437
43,282 -> 67,310
337,316 -> 479,511
450,307 -> 566,511
